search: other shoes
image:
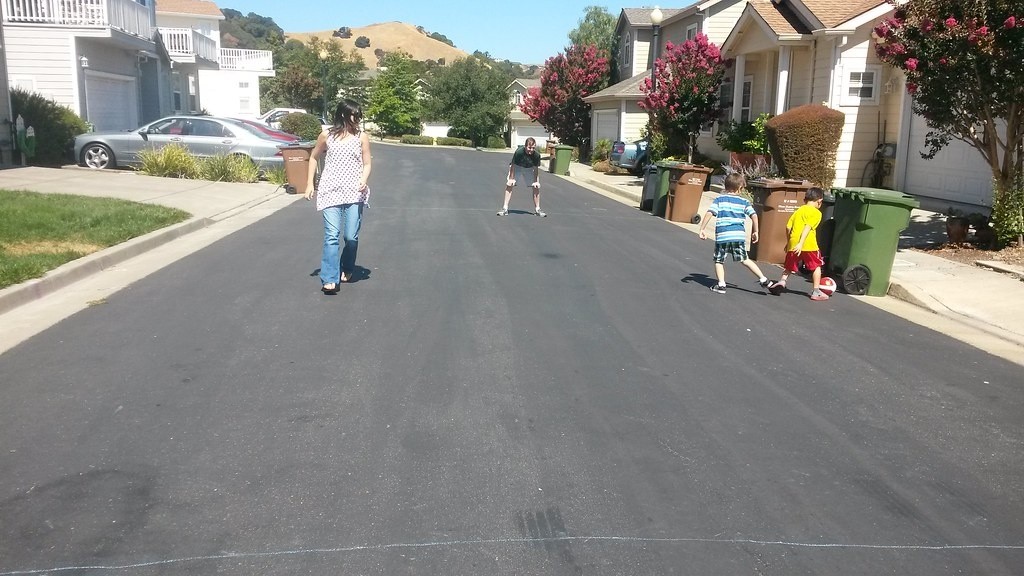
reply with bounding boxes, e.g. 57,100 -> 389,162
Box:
323,283 -> 335,291
341,271 -> 352,281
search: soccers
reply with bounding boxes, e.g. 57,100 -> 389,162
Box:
819,277 -> 837,295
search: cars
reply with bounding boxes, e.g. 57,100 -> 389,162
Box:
72,110 -> 334,183
609,135 -> 657,175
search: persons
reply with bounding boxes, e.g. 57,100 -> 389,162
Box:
497,137 -> 547,217
304,99 -> 372,292
769,187 -> 829,300
699,173 -> 773,293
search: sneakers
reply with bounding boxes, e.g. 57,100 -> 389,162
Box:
811,290 -> 829,300
710,283 -> 726,293
769,281 -> 786,294
534,208 -> 547,217
497,209 -> 508,216
757,278 -> 773,288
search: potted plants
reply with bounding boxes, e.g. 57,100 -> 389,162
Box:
942,207 -> 987,245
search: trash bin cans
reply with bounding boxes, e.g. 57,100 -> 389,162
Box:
280,141 -> 321,194
747,177 -> 814,264
652,161 -> 689,216
816,187 -> 920,297
640,164 -> 657,211
664,162 -> 714,224
549,144 -> 573,176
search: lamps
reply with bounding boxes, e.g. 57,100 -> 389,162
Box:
884,77 -> 898,95
79,54 -> 89,68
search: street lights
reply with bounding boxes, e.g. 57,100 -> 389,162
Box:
320,52 -> 328,122
